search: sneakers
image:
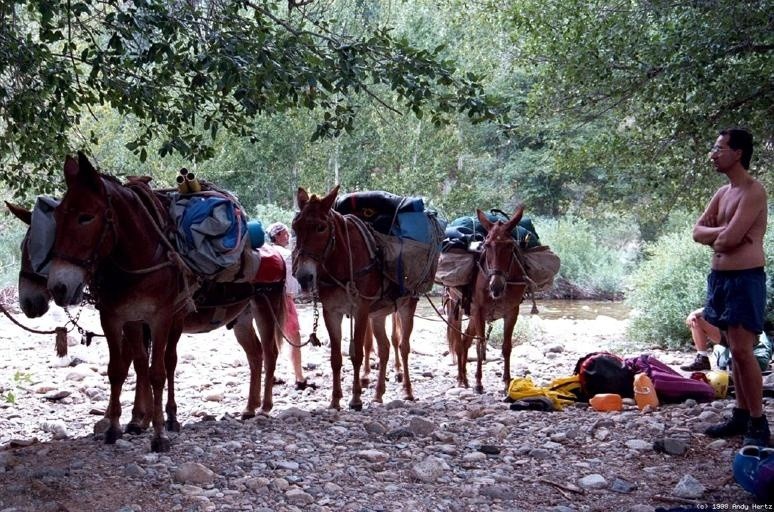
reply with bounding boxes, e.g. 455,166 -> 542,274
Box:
703,407 -> 749,439
743,414 -> 773,447
680,353 -> 711,371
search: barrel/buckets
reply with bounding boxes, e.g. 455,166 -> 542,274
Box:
634,371 -> 659,413
589,392 -> 622,412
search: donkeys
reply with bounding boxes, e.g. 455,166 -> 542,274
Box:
444,205 -> 531,397
4,149 -> 290,454
292,183 -> 420,412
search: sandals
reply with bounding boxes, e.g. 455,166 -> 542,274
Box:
271,376 -> 321,391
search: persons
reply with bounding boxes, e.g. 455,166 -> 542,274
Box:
692,126 -> 771,444
264,221 -> 321,390
680,306 -> 761,370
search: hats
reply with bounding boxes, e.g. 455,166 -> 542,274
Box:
267,223 -> 287,236
